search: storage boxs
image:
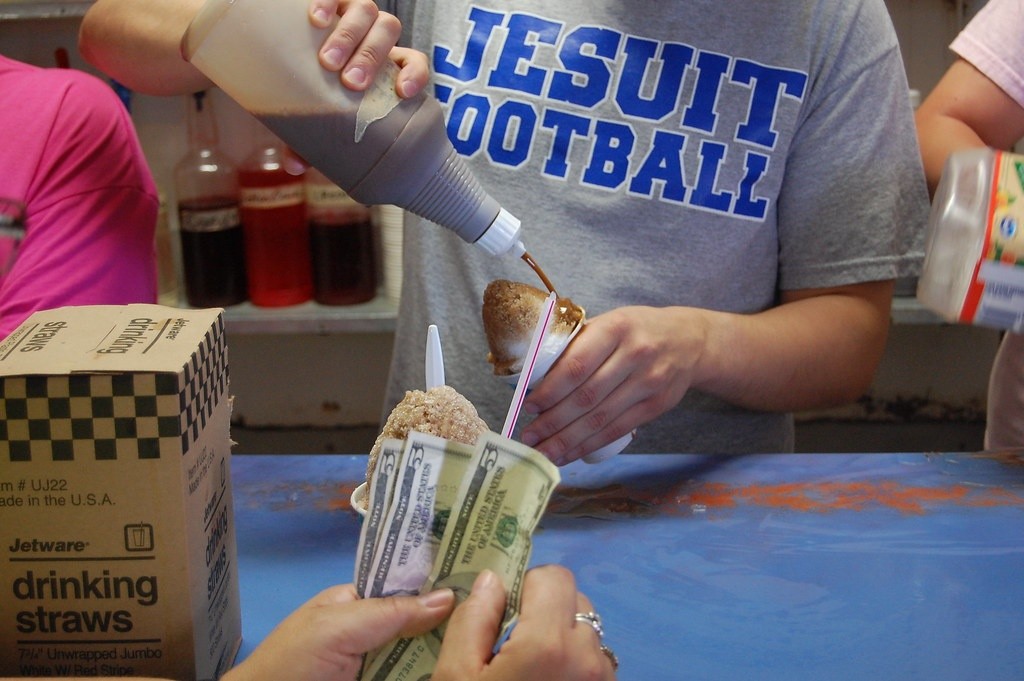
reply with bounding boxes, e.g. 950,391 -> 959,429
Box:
0,302 -> 243,681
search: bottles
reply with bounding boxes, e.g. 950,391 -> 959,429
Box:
304,159 -> 376,307
172,85 -> 247,309
917,147 -> 1023,333
179,0 -> 526,261
238,113 -> 312,308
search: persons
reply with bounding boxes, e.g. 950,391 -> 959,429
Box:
0,54 -> 161,343
80,1 -> 930,457
1,563 -> 618,681
914,0 -> 1024,453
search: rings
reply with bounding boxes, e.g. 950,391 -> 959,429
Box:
601,644 -> 619,671
575,612 -> 605,638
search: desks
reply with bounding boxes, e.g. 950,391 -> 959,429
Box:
229,454 -> 1024,681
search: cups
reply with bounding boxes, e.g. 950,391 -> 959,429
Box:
500,307 -> 637,464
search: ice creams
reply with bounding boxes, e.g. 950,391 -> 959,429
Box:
483,278 -> 582,377
359,385 -> 488,511
